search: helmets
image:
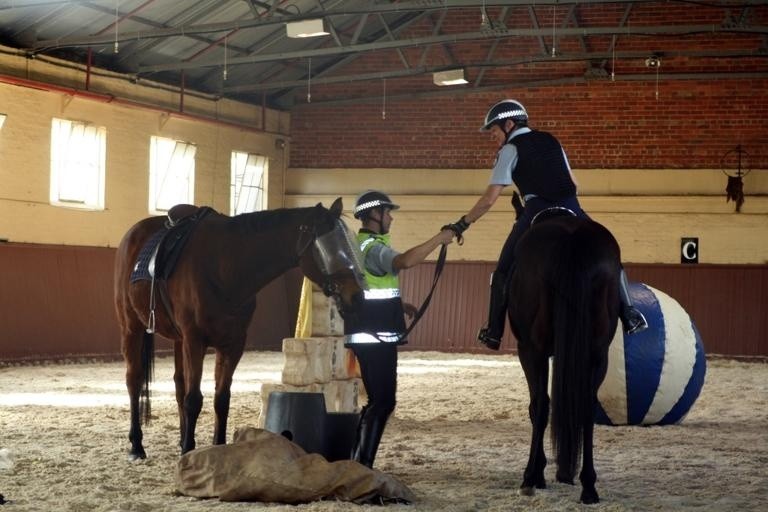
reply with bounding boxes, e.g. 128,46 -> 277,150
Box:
353,191 -> 400,219
479,98 -> 529,132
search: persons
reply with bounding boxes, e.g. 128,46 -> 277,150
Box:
441,98 -> 649,351
343,191 -> 454,470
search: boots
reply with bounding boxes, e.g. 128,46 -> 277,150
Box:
617,269 -> 645,335
351,407 -> 391,467
478,270 -> 511,351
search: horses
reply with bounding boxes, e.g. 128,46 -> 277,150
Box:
506,190 -> 622,507
113,196 -> 366,461
